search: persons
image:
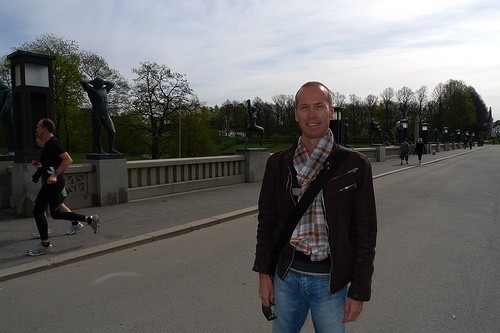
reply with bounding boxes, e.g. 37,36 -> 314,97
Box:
470,139 -> 473,149
0,78 -> 13,156
243,99 -> 264,149
413,138 -> 427,165
31,133 -> 83,238
394,118 -> 403,143
78,77 -> 119,154
481,139 -> 484,147
400,138 -> 409,165
24,119 -> 100,256
431,128 -> 441,142
478,139 -> 480,147
493,140 -> 495,145
343,118 -> 349,145
443,130 -> 472,142
250,82 -> 378,333
372,119 -> 385,144
464,138 -> 468,149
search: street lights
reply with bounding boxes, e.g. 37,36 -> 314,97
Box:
329,107 -> 344,145
456,128 -> 461,149
399,118 -> 409,143
420,122 -> 431,154
442,126 -> 450,150
6,50 -> 58,218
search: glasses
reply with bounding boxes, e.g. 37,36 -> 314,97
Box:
262,301 -> 277,321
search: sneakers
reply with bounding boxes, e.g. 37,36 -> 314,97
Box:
86,214 -> 99,234
66,221 -> 84,235
25,242 -> 54,255
31,226 -> 52,235
